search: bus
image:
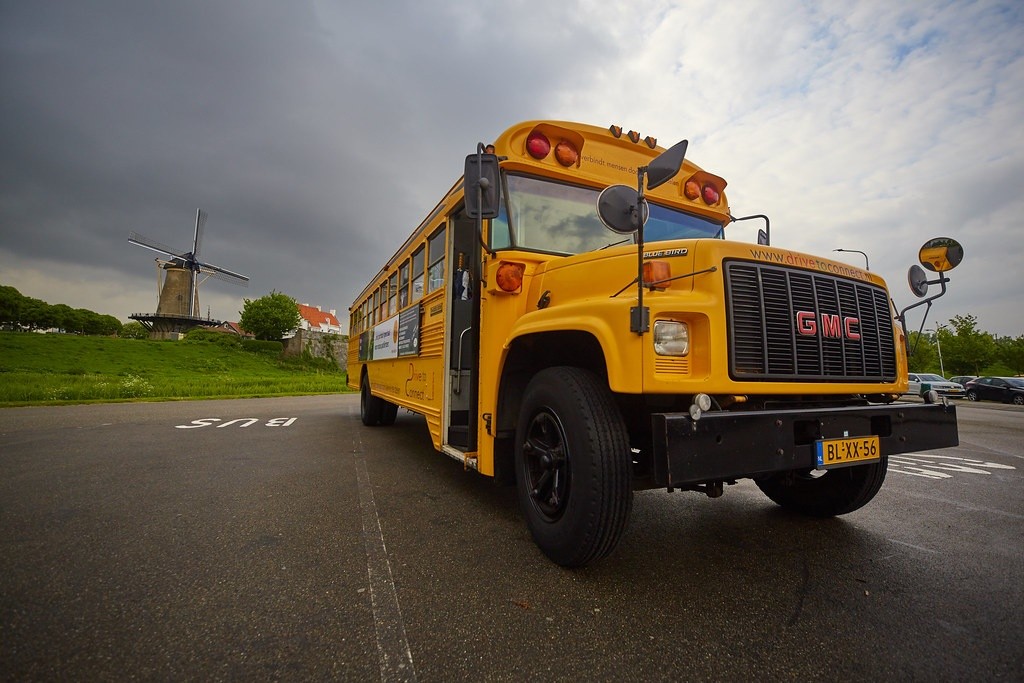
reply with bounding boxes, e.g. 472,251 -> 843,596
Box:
345,119 -> 965,574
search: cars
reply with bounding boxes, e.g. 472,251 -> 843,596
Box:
906,373 -> 966,398
949,375 -> 987,390
965,376 -> 1024,405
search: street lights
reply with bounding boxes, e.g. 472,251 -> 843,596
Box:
925,329 -> 945,378
